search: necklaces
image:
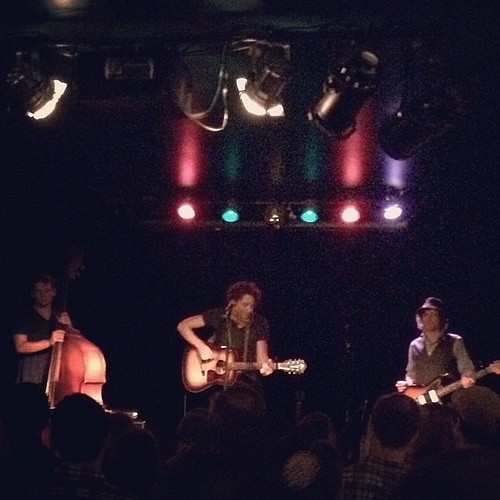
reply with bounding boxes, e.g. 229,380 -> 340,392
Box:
425,337 -> 439,345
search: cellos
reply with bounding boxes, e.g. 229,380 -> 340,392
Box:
45,258 -> 107,410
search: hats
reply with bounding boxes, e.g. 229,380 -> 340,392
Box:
416,297 -> 448,313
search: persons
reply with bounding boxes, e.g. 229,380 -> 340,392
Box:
0,382 -> 500,500
177,279 -> 274,412
396,296 -> 500,404
7,275 -> 71,382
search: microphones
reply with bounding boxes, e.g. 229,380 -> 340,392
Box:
225,300 -> 236,311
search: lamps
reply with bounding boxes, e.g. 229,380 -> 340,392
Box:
305,40 -> 468,160
176,36 -> 291,134
6,45 -> 70,121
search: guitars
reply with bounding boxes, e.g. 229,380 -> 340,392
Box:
404,360 -> 500,407
181,342 -> 307,393
